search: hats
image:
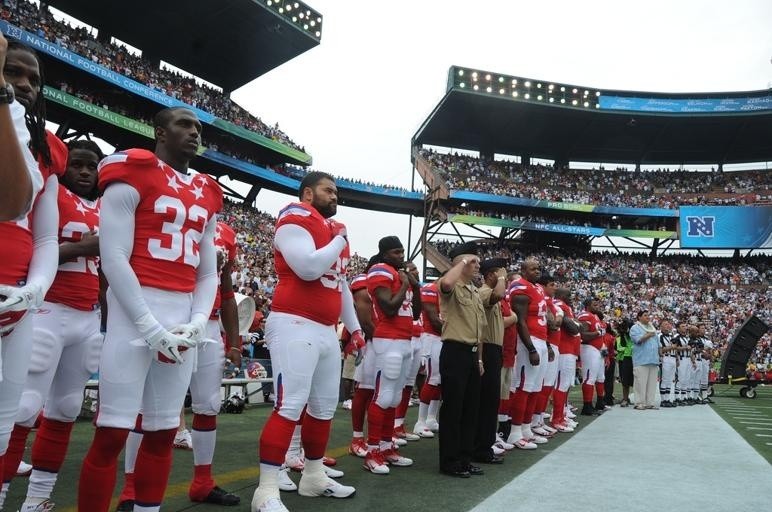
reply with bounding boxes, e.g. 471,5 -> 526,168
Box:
478,256 -> 508,274
259,317 -> 267,323
378,235 -> 404,253
448,240 -> 478,262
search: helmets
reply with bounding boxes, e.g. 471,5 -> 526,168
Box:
222,391 -> 247,414
247,361 -> 267,379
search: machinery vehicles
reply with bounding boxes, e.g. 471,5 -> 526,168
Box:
706,372 -> 772,399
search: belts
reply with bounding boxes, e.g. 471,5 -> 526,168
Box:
664,354 -> 676,357
443,340 -> 478,353
696,359 -> 702,361
679,356 -> 691,360
483,343 -> 503,352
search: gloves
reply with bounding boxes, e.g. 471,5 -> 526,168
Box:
168,322 -> 199,355
144,327 -> 197,367
0,281 -> 44,338
596,324 -> 607,337
350,329 -> 367,368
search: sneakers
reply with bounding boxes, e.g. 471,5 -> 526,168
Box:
286,455 -> 305,471
15,461 -> 33,476
189,481 -> 241,507
172,429 -> 193,452
322,457 -> 338,466
264,395 -> 275,403
391,429 -> 408,446
250,486 -> 291,512
379,446 -> 413,467
298,470 -> 356,500
277,466 -> 297,492
323,463 -> 345,478
441,401 -> 580,479
349,440 -> 369,458
363,450 -> 390,475
413,420 -> 439,439
581,394 -> 715,416
393,427 -> 420,441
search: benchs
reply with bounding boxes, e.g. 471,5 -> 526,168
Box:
84,377 -> 273,402
